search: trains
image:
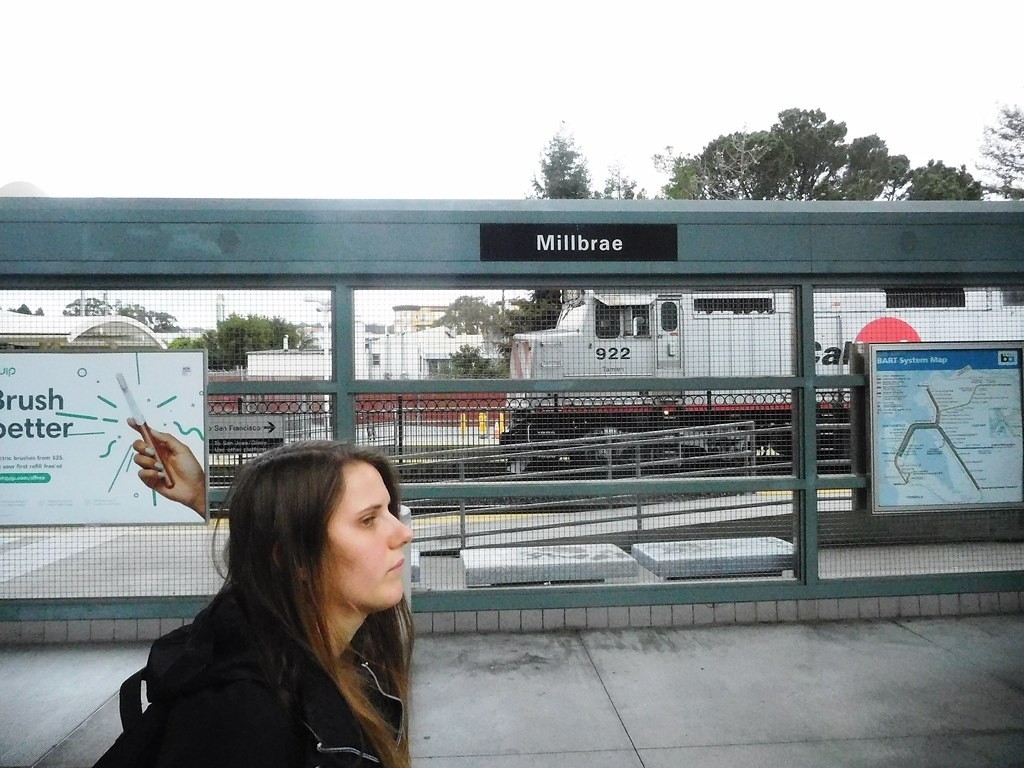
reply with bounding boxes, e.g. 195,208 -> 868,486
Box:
494,280 -> 1024,480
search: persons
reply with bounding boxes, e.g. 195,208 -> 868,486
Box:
86,440 -> 413,768
127,417 -> 207,521
